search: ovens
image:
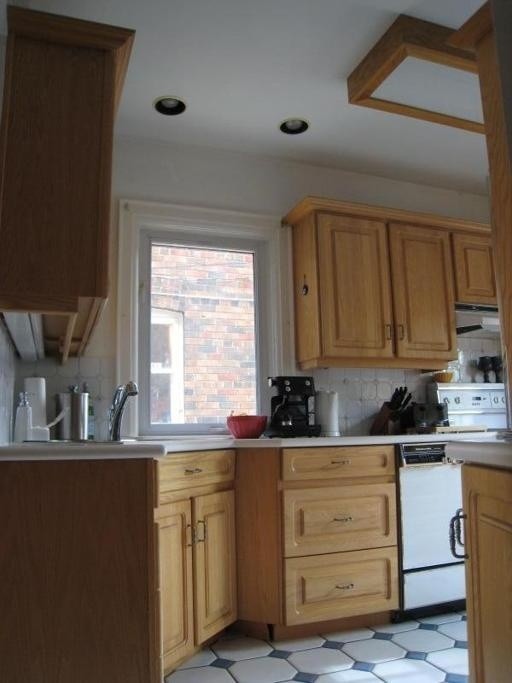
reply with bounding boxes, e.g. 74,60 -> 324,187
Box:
399,442 -> 468,573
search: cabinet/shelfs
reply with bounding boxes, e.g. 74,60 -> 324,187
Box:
237,445 -> 402,643
448,464 -> 512,683
1,448 -> 240,682
283,196 -> 459,371
450,221 -> 500,305
1,1 -> 136,358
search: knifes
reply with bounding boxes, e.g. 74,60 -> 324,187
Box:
388,382 -> 412,412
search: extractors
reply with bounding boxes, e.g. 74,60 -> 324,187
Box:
455,308 -> 500,342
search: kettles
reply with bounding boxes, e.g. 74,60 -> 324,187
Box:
313,389 -> 342,436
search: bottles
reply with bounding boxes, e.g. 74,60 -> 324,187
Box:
81,380 -> 97,440
15,391 -> 32,442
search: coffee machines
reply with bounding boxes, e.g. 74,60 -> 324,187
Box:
266,374 -> 321,437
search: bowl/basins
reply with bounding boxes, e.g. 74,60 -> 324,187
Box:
226,415 -> 269,439
434,372 -> 452,382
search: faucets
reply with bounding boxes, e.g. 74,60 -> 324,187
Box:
107,381 -> 139,440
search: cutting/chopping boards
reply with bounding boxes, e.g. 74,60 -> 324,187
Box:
416,425 -> 487,433
